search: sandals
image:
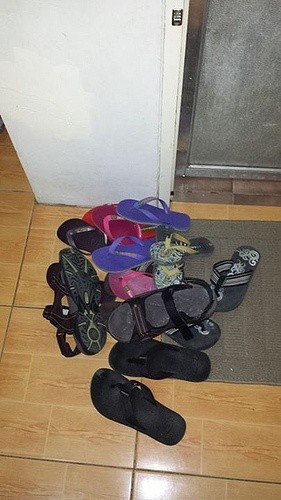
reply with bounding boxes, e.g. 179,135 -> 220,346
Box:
43,244 -> 105,363
107,276 -> 216,346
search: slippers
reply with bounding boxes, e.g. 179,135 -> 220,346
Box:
105,338 -> 211,385
151,232 -> 213,266
89,367 -> 187,446
207,244 -> 261,313
91,234 -> 157,273
116,197 -> 190,232
138,222 -> 157,239
153,261 -> 185,290
165,319 -> 220,351
91,204 -> 143,243
56,217 -> 112,256
106,268 -> 154,300
